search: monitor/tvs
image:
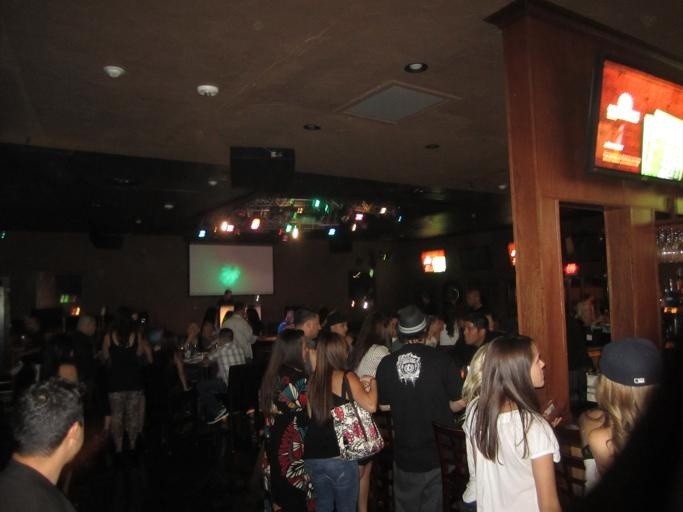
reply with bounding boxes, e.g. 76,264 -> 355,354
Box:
585,54 -> 682,190
418,248 -> 448,274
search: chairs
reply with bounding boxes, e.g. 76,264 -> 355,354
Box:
216,364 -> 261,429
369,423 -> 400,512
432,420 -> 467,511
552,427 -> 585,512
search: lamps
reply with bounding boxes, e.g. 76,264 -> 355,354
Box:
192,191 -> 402,241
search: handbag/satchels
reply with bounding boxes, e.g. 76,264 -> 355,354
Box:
326,397 -> 388,462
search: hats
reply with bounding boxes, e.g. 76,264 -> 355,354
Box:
395,303 -> 432,340
597,334 -> 662,389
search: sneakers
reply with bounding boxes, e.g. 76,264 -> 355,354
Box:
206,408 -> 231,426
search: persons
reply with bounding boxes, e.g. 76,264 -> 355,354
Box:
258,288 -> 563,512
177,290 -> 276,424
2,285 -> 153,510
563,293 -> 681,511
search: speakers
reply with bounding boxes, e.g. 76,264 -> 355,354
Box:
105,174 -> 211,234
231,146 -> 267,187
1,144 -> 74,209
268,148 -> 295,175
